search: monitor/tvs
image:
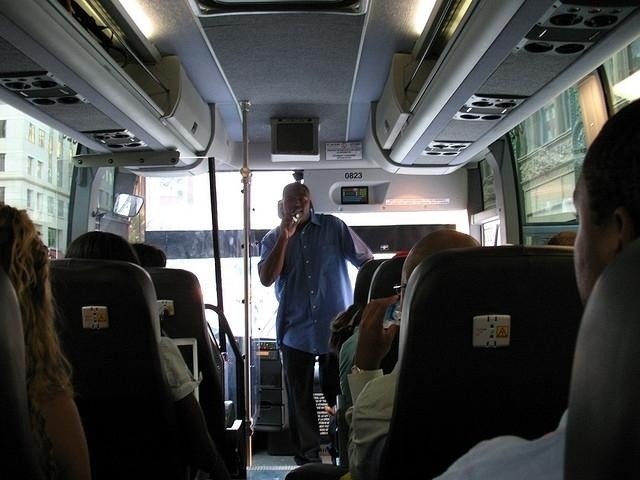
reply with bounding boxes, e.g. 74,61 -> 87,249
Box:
269,117 -> 321,161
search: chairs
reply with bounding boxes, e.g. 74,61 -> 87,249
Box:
344,255 -> 640,477
1,257 -> 234,480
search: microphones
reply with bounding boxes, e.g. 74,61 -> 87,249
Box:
289,208 -> 301,225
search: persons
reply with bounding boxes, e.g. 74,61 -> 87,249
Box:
1,203 -> 231,479
257,182 -> 374,464
322,100 -> 639,480
